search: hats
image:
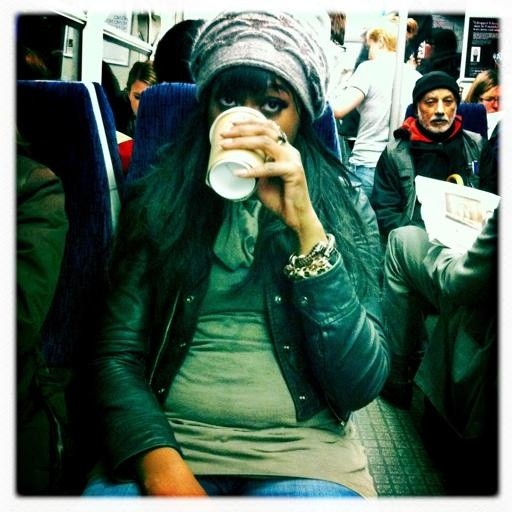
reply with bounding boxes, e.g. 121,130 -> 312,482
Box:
187,3 -> 331,127
412,70 -> 461,106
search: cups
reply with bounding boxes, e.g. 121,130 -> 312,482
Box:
206,106 -> 267,202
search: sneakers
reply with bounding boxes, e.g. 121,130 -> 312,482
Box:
379,379 -> 413,411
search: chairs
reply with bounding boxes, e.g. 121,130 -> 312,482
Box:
402,103 -> 488,153
119,80 -> 343,196
16,80 -> 121,365
